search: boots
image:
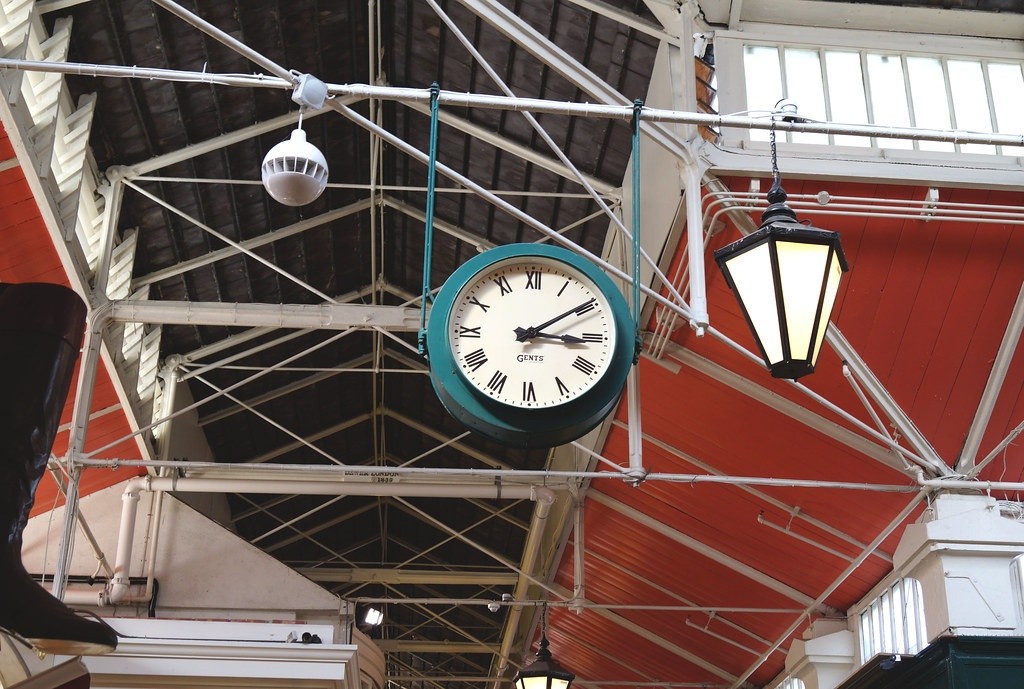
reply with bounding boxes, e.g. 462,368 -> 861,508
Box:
0,280 -> 120,658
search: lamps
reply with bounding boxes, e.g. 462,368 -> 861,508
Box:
711,125 -> 848,377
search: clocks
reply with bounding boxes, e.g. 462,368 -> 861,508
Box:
417,82 -> 646,451
